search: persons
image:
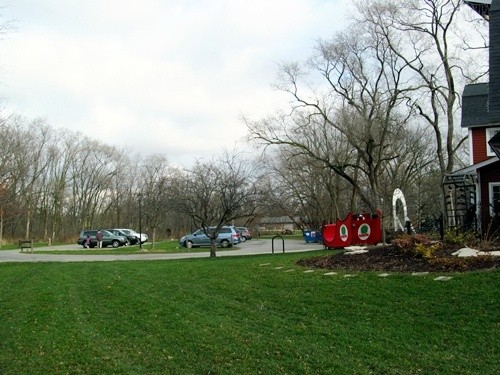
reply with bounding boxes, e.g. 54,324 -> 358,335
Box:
300,220 -> 314,237
95,230 -> 103,249
83,234 -> 92,248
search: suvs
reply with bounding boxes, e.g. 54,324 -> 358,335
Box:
180,225 -> 253,248
76,228 -> 149,248
